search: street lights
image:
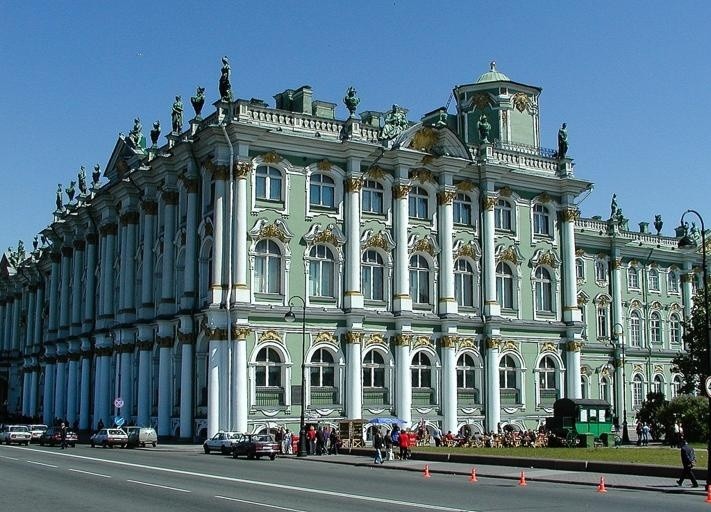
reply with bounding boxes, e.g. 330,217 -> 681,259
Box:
677,208 -> 711,375
284,294 -> 310,456
611,321 -> 633,444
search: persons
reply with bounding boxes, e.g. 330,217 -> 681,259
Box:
219,57 -> 232,100
641,421 -> 651,446
671,420 -> 679,448
98,419 -> 104,432
281,426 -> 538,464
171,96 -> 184,133
637,421 -> 643,446
129,118 -> 142,145
379,104 -> 408,140
676,440 -> 698,488
559,123 -> 568,159
60,423 -> 69,449
78,166 -> 87,194
56,184 -> 63,210
17,241 -> 25,265
437,113 -> 448,126
691,221 -> 700,236
611,193 -> 617,215
674,422 -> 684,448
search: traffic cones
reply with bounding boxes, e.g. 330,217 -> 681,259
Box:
469,466 -> 479,481
518,470 -> 529,485
598,476 -> 608,492
704,485 -> 711,503
423,464 -> 433,477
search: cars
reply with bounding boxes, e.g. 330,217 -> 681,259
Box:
201,431 -> 250,454
89,427 -> 129,448
232,433 -> 282,460
27,424 -> 49,441
123,426 -> 143,436
0,423 -> 32,447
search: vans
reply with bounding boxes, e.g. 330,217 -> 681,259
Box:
38,425 -> 79,448
128,427 -> 160,447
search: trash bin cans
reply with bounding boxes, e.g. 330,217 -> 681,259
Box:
580,433 -> 594,448
602,432 -> 616,448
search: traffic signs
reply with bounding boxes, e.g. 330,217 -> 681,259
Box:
705,376 -> 711,397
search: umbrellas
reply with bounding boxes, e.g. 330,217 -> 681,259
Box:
368,417 -> 407,424
